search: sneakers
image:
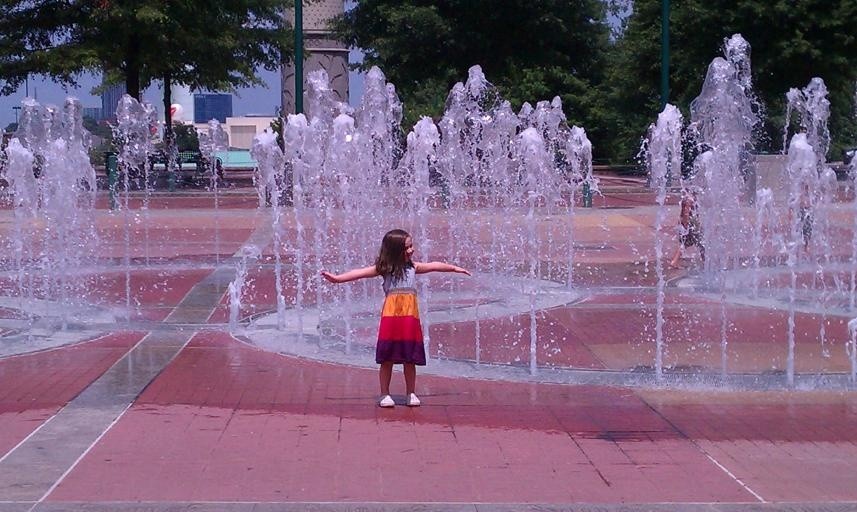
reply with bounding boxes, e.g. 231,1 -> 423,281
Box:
379,395 -> 395,407
406,393 -> 420,405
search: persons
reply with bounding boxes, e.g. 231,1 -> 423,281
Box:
319,229 -> 471,407
789,182 -> 814,253
670,187 -> 706,269
